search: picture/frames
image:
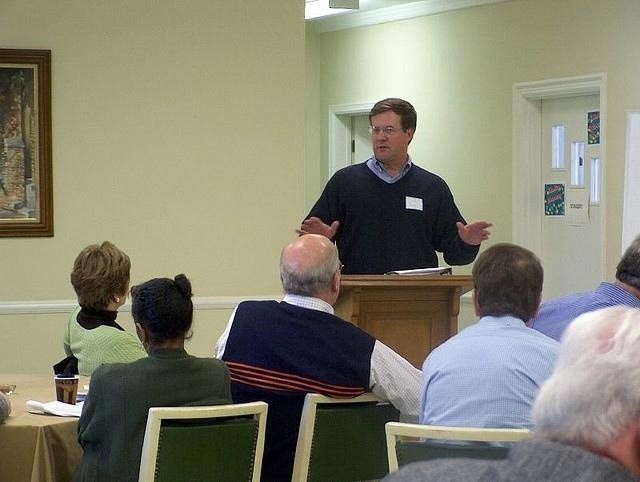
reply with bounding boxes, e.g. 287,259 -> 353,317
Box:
0,49 -> 55,238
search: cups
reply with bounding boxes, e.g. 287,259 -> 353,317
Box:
53,373 -> 80,406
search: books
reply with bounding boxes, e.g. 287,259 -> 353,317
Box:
380,264 -> 453,277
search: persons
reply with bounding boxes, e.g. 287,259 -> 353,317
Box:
530,230 -> 639,344
62,240 -> 149,376
293,98 -> 494,276
416,243 -> 563,448
213,232 -> 423,480
374,302 -> 640,481
71,273 -> 234,482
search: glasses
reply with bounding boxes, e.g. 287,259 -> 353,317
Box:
367,125 -> 402,136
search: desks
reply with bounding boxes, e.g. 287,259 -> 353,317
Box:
0,374 -> 93,481
